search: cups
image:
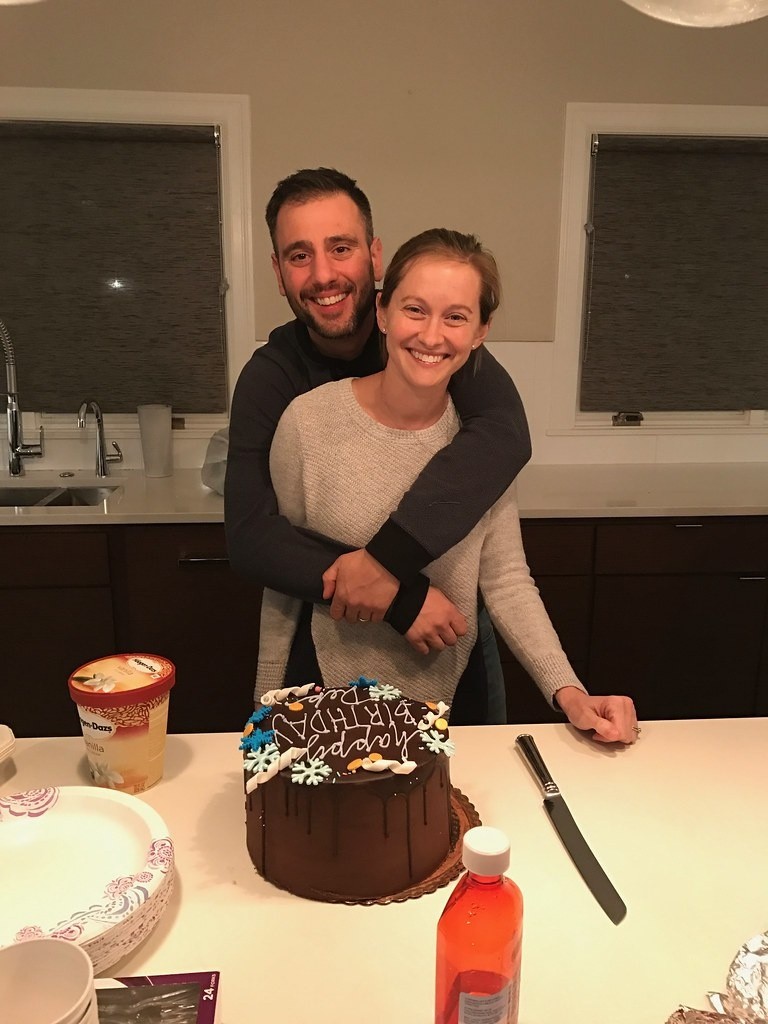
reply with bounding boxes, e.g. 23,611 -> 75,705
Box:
137,404 -> 172,478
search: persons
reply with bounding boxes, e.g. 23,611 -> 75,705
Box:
220,165 -> 535,725
252,225 -> 642,746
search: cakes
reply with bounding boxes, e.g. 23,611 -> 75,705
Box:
238,676 -> 455,904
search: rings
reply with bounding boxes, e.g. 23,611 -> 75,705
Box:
631,725 -> 641,733
358,617 -> 370,623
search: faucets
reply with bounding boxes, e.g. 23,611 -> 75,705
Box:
76,398 -> 108,478
2,320 -> 24,478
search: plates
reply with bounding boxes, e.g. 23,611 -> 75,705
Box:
0,786 -> 176,976
0,724 -> 16,764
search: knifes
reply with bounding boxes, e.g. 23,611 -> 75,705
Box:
515,734 -> 627,925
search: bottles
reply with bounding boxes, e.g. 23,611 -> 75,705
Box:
435,826 -> 524,1024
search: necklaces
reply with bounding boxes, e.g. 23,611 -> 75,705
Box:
374,374 -> 452,457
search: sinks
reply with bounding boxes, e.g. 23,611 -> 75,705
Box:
0,484 -> 56,507
49,483 -> 114,510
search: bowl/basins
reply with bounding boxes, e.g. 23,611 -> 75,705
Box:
0,938 -> 98,1024
